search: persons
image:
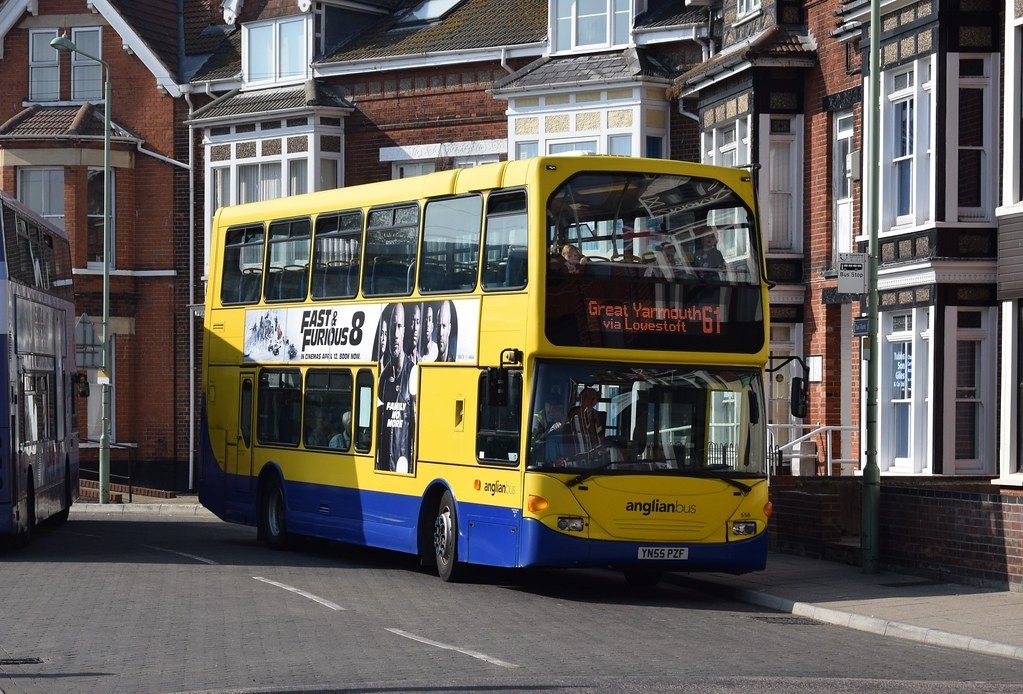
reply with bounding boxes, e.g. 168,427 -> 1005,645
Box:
528,384 -> 574,466
644,242 -> 689,278
694,223 -> 727,281
563,388 -> 606,458
329,411 -> 357,448
371,301 -> 455,474
550,245 -> 592,274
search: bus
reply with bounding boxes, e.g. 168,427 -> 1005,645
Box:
0,186 -> 91,548
193,150 -> 814,587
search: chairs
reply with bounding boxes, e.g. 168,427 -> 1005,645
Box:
278,265 -> 307,300
258,267 -> 283,300
301,263 -> 326,297
589,252 -> 657,276
239,269 -> 263,302
323,261 -> 350,296
505,245 -> 528,287
347,256 -> 508,295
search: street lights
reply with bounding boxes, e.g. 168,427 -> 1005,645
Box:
53,35 -> 114,503
